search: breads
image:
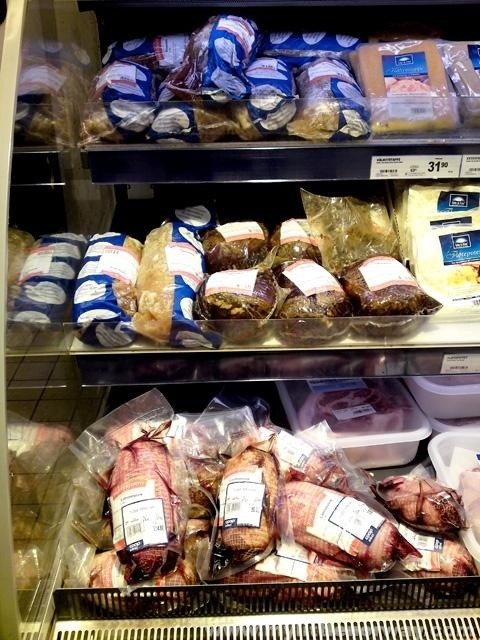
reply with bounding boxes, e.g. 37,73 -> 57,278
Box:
348,35 -> 480,137
395,182 -> 479,320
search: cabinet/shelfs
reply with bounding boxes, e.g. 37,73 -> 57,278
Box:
1,1 -> 480,640
